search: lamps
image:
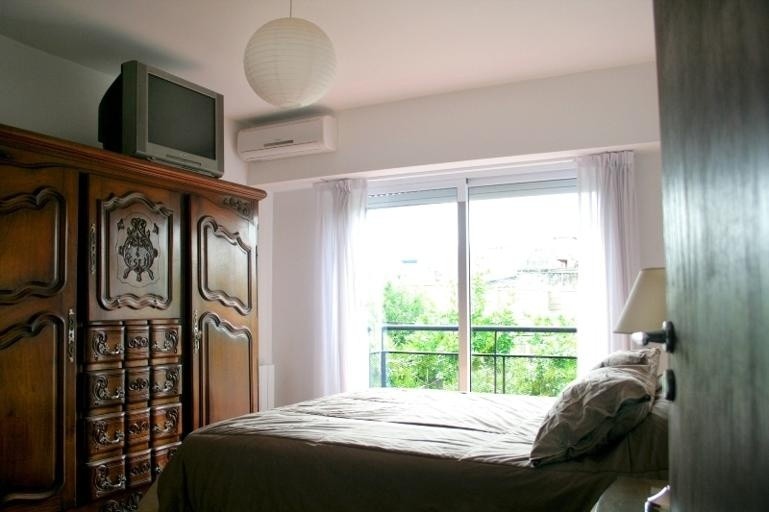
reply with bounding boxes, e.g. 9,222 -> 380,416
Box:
611,267 -> 668,334
243,2 -> 339,110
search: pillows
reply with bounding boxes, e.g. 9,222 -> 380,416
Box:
530,365 -> 659,467
591,347 -> 661,379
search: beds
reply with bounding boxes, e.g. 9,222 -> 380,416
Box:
139,390 -> 668,511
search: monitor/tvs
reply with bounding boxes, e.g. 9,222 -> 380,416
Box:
98,60 -> 225,179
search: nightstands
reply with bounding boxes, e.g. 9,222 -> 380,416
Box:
590,474 -> 667,511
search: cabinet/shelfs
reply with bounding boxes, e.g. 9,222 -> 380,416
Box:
81,168 -> 185,512
0,144 -> 81,512
184,186 -> 269,436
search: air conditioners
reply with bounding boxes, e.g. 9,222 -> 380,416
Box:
236,115 -> 338,163
